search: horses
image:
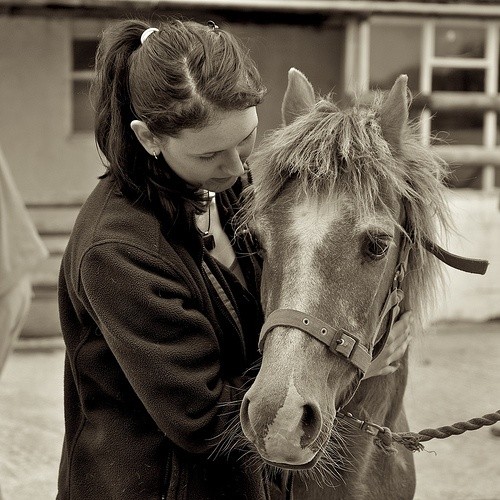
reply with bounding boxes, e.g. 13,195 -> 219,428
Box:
231,61 -> 467,500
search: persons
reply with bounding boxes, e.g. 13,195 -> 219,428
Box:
56,20 -> 414,500
0,149 -> 48,374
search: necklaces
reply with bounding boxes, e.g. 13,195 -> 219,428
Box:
195,189 -> 216,252
